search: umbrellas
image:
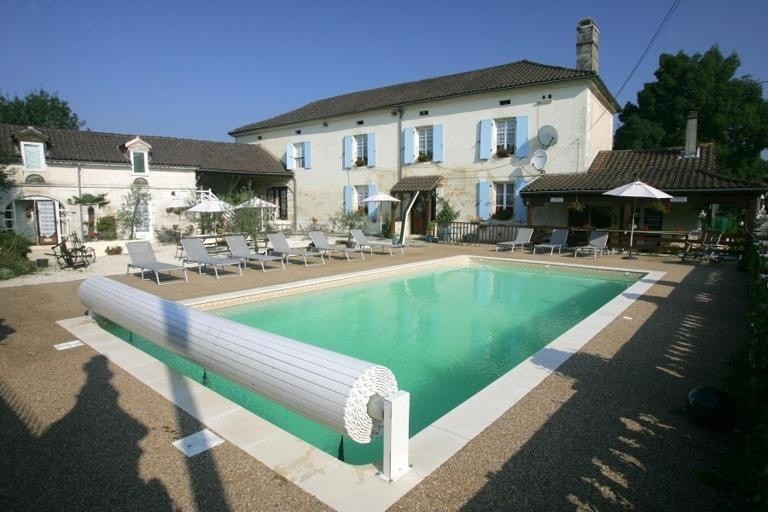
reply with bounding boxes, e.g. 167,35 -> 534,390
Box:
601,179 -> 674,260
361,190 -> 402,231
231,196 -> 280,209
187,198 -> 234,247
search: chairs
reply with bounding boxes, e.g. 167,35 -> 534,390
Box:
222,235 -> 286,273
268,229 -> 409,268
181,237 -> 244,280
121,241 -> 191,286
533,228 -> 570,255
682,230 -> 725,263
495,228 -> 535,254
574,231 -> 612,260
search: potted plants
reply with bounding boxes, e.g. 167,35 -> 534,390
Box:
437,202 -> 458,242
424,222 -> 434,243
